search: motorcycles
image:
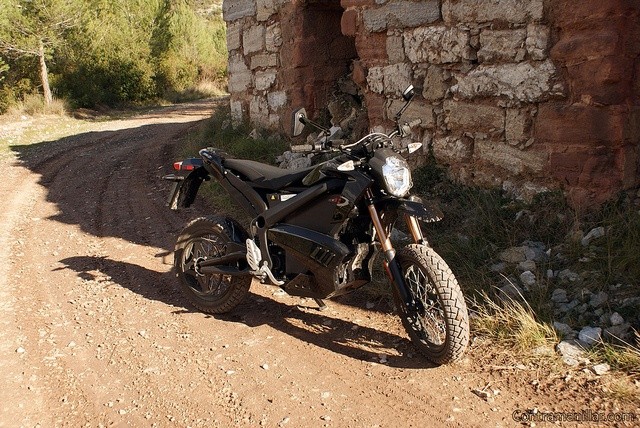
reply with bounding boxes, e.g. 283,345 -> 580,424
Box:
162,84 -> 470,366
